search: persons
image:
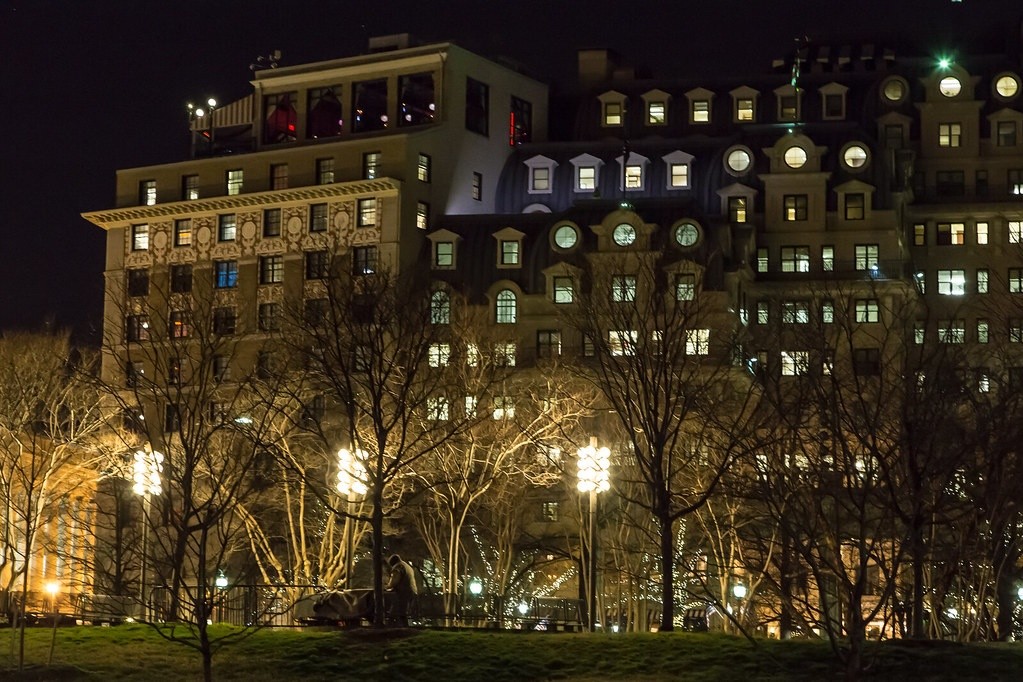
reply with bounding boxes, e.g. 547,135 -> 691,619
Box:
386,555 -> 417,626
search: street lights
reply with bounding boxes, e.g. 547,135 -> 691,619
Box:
734,580 -> 747,624
132,443 -> 165,620
469,579 -> 483,628
336,448 -> 369,590
215,573 -> 228,623
46,581 -> 60,612
574,435 -> 612,632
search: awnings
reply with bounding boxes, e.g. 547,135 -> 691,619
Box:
190,94 -> 253,157
